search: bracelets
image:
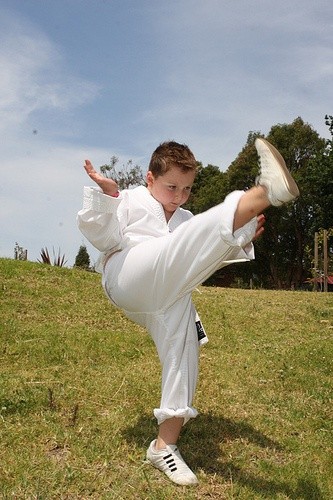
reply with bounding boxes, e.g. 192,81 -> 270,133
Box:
112,192 -> 119,198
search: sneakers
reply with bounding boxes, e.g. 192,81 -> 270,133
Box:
146,438 -> 198,485
255,137 -> 299,207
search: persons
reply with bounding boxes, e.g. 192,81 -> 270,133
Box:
77,139 -> 299,487
